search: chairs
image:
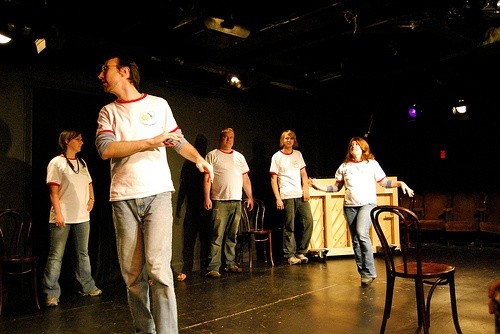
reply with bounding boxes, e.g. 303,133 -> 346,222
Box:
370,206 -> 461,334
0,208 -> 41,316
240,199 -> 275,274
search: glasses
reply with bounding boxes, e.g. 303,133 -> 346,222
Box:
102,65 -> 120,71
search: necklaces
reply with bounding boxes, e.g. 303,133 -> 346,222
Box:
66,157 -> 80,174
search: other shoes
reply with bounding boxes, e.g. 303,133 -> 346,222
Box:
225,265 -> 242,271
79,286 -> 102,296
209,270 -> 221,276
47,298 -> 60,305
360,277 -> 374,288
288,254 -> 308,265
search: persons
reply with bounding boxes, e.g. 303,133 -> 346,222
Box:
98,57 -> 214,333
310,138 -> 414,287
41,130 -> 102,306
269,130 -> 313,264
204,126 -> 253,277
